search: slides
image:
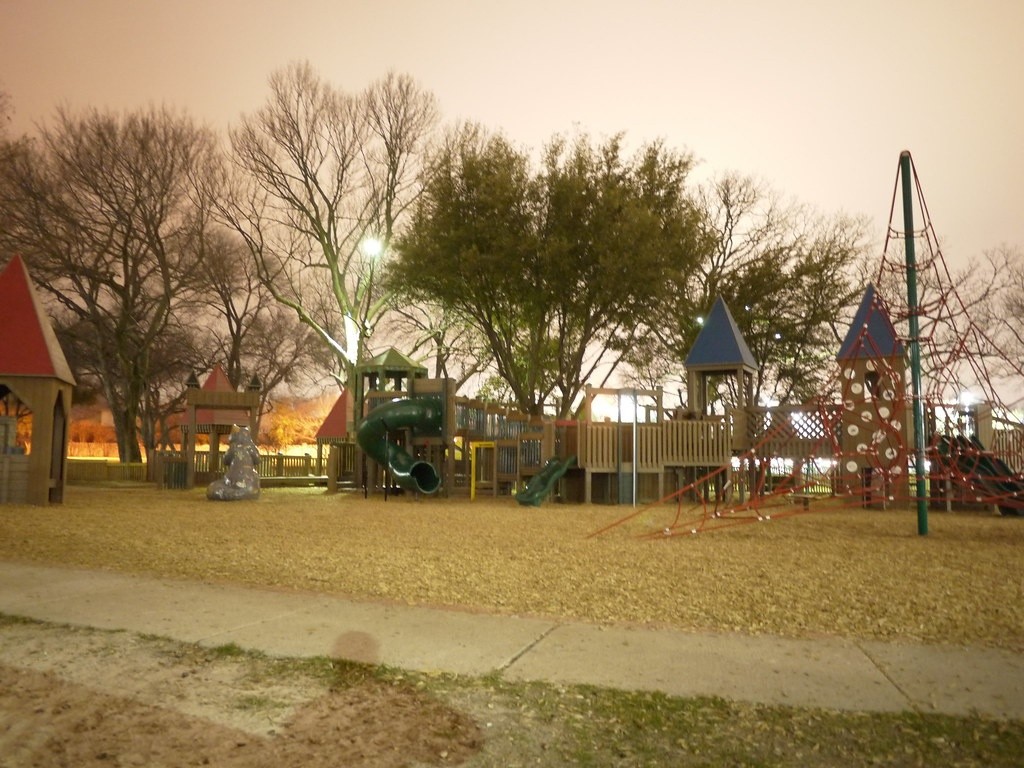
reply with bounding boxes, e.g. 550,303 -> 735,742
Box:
933,434 -> 1024,508
517,453 -> 577,508
356,398 -> 446,496
